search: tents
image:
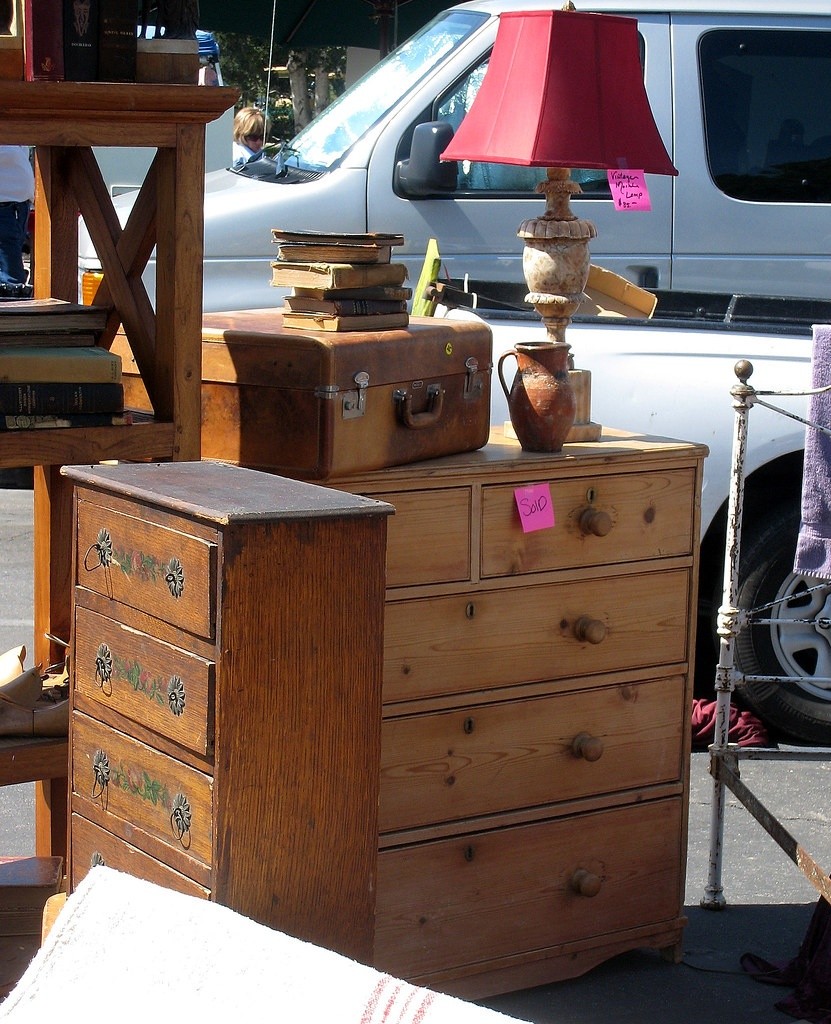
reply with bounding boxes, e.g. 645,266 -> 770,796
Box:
137,25 -> 223,86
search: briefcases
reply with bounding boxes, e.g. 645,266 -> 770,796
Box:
112,306 -> 500,479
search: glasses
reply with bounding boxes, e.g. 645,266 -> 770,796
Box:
244,133 -> 266,143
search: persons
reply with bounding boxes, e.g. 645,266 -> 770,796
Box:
0,146 -> 35,284
233,107 -> 270,166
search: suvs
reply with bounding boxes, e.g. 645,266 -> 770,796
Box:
78,0 -> 831,316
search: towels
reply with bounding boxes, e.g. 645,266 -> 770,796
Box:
792,323 -> 831,581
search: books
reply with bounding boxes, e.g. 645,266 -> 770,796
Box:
270,230 -> 413,332
26,0 -> 137,84
0,298 -> 132,429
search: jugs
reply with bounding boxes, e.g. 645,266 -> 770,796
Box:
498,341 -> 576,453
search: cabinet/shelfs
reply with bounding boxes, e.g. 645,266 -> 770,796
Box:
0,85 -> 696,1004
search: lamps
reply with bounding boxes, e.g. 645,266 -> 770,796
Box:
435,10 -> 678,448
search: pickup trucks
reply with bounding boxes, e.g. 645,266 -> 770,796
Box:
421,268 -> 831,748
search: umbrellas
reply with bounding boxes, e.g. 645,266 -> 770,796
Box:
136,0 -> 462,59
